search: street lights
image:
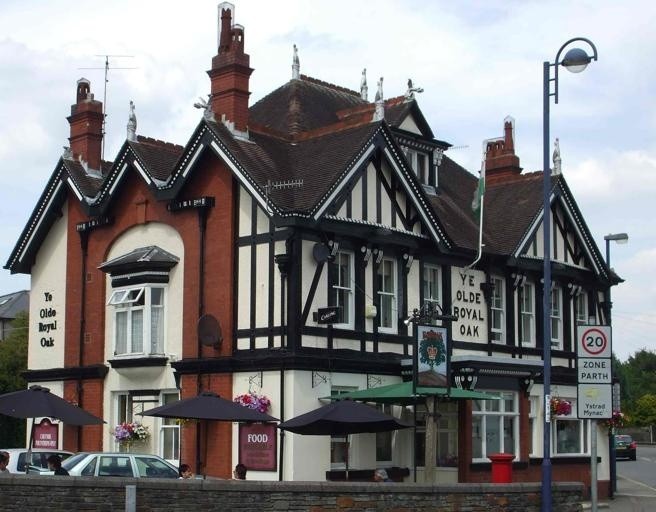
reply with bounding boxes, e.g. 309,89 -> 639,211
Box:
603,230 -> 627,273
539,39 -> 599,511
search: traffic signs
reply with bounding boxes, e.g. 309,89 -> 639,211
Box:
578,326 -> 612,359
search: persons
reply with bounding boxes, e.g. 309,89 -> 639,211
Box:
46,454 -> 70,476
177,465 -> 195,480
374,468 -> 393,482
0,451 -> 12,476
232,464 -> 247,480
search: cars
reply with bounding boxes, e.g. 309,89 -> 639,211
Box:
27,451 -> 222,480
0,448 -> 75,475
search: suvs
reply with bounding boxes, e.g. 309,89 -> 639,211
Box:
615,433 -> 637,461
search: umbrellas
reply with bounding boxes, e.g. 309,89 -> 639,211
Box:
275,398 -> 417,482
135,393 -> 280,479
0,384 -> 107,475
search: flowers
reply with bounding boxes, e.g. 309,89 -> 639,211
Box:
106,420 -> 151,450
550,396 -> 574,418
598,410 -> 632,438
233,391 -> 273,414
